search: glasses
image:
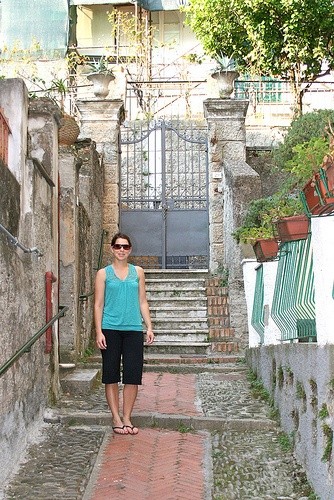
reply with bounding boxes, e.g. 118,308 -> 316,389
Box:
112,244 -> 130,250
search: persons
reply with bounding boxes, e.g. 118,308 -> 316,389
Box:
93,232 -> 156,436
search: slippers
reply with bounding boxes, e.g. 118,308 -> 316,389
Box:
112,425 -> 128,435
126,426 -> 139,435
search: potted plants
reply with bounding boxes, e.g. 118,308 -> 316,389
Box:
240,227 -> 278,261
210,49 -> 240,98
86,55 -> 115,99
290,130 -> 334,215
271,192 -> 309,244
